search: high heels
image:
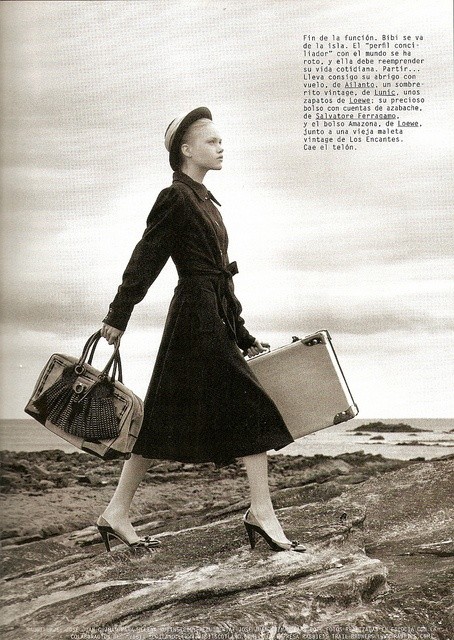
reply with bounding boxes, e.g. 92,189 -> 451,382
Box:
96,515 -> 163,551
243,506 -> 308,552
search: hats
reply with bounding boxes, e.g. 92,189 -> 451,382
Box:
164,107 -> 213,170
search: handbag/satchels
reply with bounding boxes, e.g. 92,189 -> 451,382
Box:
33,331 -> 121,440
23,333 -> 144,463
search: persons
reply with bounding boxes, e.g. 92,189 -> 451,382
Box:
95,106 -> 307,553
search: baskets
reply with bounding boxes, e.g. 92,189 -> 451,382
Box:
243,330 -> 360,439
